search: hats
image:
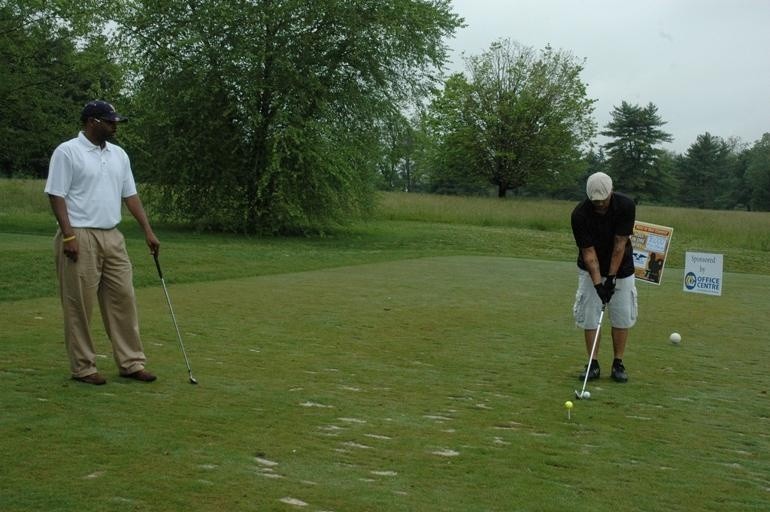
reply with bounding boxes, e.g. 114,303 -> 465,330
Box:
81,100 -> 130,126
587,171 -> 614,202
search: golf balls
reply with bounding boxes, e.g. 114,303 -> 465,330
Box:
670,333 -> 681,344
565,401 -> 572,408
584,391 -> 590,398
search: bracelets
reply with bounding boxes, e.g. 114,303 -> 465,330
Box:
60,234 -> 76,242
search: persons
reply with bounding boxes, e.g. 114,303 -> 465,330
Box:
43,100 -> 161,389
571,172 -> 636,383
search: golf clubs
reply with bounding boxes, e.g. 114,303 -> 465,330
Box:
575,305 -> 605,399
154,254 -> 197,384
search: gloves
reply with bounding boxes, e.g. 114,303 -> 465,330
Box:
593,274 -> 619,306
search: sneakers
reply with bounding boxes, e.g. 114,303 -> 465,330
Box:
611,363 -> 629,383
579,363 -> 601,381
123,369 -> 157,383
82,372 -> 108,385
